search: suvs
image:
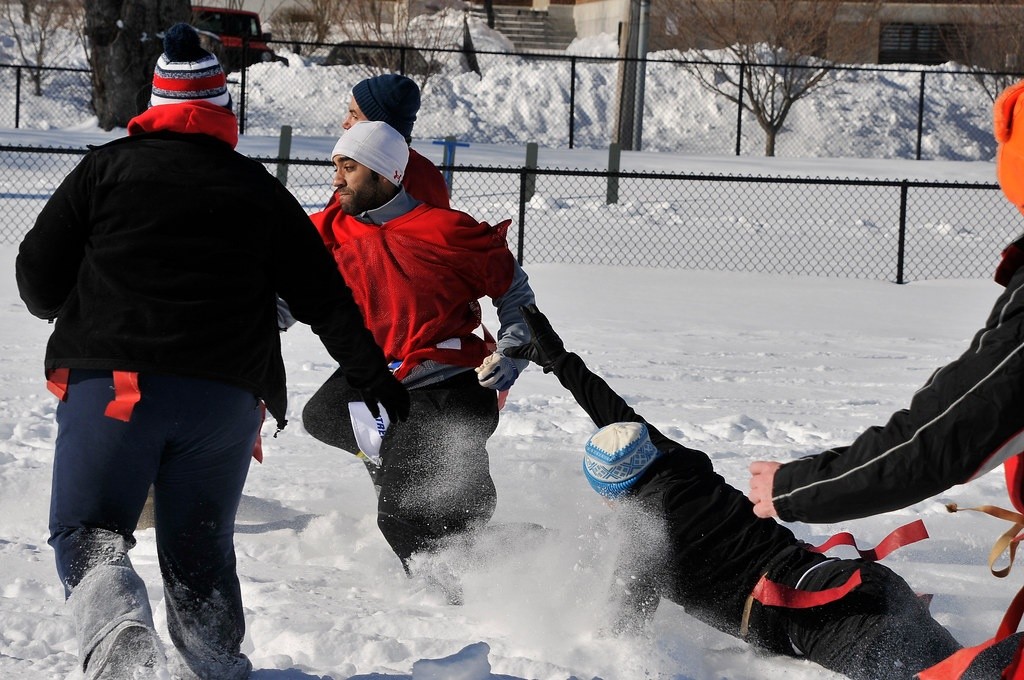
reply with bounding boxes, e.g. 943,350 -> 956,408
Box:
191,5 -> 289,79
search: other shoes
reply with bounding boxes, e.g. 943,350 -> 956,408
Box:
237,654 -> 252,680
85,619 -> 154,680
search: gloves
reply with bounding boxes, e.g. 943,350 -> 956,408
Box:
348,364 -> 410,425
475,355 -> 517,391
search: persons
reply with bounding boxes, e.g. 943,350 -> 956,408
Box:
503,302 -> 965,680
277,73 -> 536,606
15,23 -> 411,680
747,76 -> 1024,680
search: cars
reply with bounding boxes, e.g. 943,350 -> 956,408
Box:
324,40 -> 445,76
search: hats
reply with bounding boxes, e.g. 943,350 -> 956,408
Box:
147,22 -> 233,114
352,74 -> 421,138
994,79 -> 1024,215
581,420 -> 657,500
332,121 -> 408,187
